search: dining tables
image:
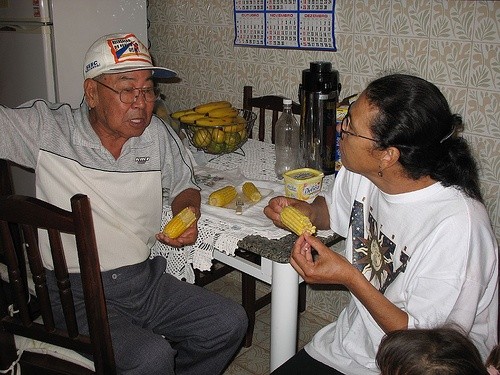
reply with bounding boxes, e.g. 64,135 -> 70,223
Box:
150,138 -> 347,373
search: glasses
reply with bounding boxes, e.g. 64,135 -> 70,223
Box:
91,78 -> 160,104
340,101 -> 381,143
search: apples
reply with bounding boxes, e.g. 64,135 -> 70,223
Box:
193,128 -> 245,153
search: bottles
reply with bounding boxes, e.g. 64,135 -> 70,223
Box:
274,99 -> 300,180
297,61 -> 342,175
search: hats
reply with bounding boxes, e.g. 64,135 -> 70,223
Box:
83,32 -> 178,82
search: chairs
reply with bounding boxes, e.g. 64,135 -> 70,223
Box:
192,86 -> 306,347
0,193 -> 166,375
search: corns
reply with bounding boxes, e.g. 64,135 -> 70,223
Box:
209,186 -> 238,206
280,205 -> 316,236
243,182 -> 262,201
162,207 -> 197,239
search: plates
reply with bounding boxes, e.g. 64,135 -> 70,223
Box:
205,186 -> 273,212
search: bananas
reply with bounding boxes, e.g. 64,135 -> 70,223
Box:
169,101 -> 247,132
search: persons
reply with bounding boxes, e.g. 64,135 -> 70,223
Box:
0,33 -> 249,375
376,319 -> 492,375
262,73 -> 499,374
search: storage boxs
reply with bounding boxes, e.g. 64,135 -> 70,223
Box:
283,167 -> 324,201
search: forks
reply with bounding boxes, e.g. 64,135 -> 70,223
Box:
235,200 -> 243,215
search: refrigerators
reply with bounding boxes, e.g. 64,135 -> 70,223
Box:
0,0 -> 152,199
